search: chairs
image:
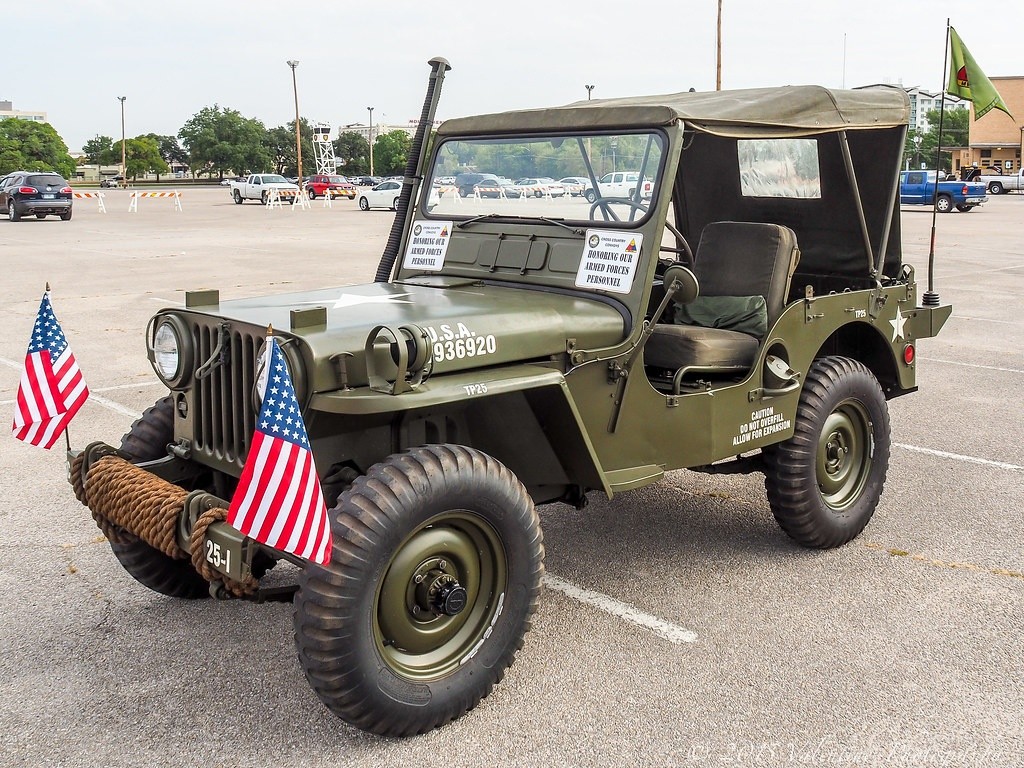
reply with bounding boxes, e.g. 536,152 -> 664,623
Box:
722,338 -> 724,343
643,220 -> 802,372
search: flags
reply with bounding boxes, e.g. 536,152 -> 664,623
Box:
947,26 -> 1016,124
225,338 -> 333,568
11,292 -> 89,450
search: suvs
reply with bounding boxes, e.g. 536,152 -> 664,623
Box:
61,55 -> 957,751
0,170 -> 74,221
306,174 -> 357,201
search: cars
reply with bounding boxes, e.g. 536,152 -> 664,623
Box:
358,179 -> 440,212
558,176 -> 591,198
100,179 -> 118,189
517,178 -> 567,199
220,179 -> 231,186
285,172 -> 513,187
473,178 -> 521,198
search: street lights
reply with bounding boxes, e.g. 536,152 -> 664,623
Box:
116,96 -> 127,187
287,60 -> 303,190
585,85 -> 594,100
367,107 -> 374,178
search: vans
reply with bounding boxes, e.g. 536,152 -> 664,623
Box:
584,171 -> 651,205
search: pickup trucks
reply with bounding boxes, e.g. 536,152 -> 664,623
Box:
982,167 -> 1024,195
229,173 -> 300,206
899,168 -> 989,213
